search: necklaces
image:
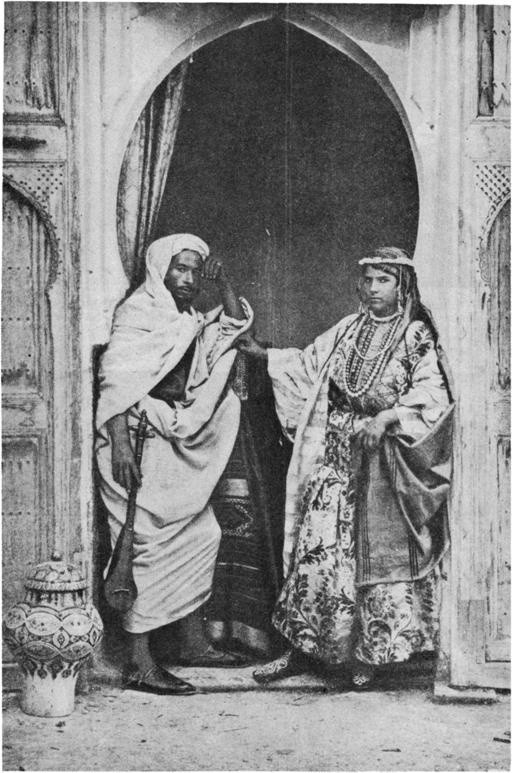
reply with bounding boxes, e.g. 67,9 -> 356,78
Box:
342,310 -> 405,400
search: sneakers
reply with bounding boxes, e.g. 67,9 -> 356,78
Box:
253,649 -> 313,683
121,663 -> 195,696
176,645 -> 252,667
351,670 -> 377,691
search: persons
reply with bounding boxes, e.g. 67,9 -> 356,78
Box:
239,246 -> 458,688
91,228 -> 257,698
